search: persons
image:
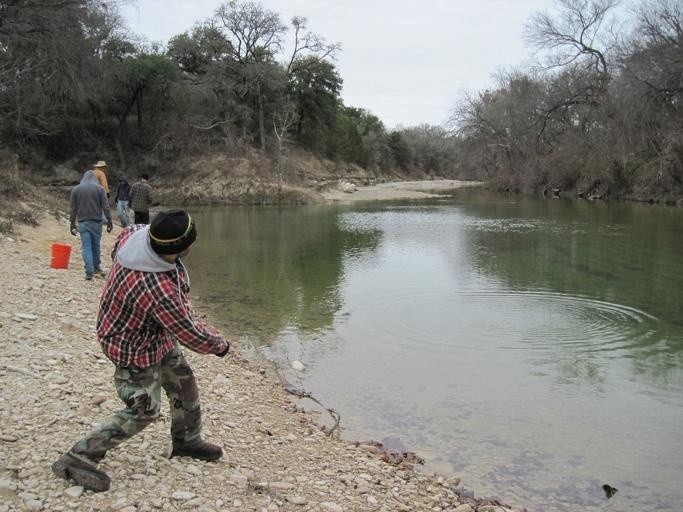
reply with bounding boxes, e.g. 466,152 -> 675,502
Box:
92,160 -> 111,225
116,174 -> 132,228
69,170 -> 114,280
129,172 -> 154,224
49,206 -> 231,494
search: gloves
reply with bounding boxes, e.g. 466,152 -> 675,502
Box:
71,224 -> 78,236
107,224 -> 113,233
215,341 -> 230,357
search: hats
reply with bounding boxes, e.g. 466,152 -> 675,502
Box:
94,161 -> 109,167
148,208 -> 197,255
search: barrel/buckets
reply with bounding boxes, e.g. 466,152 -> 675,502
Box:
50,243 -> 72,269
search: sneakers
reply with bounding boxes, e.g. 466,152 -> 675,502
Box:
50,453 -> 110,492
86,270 -> 107,280
172,436 -> 223,461
